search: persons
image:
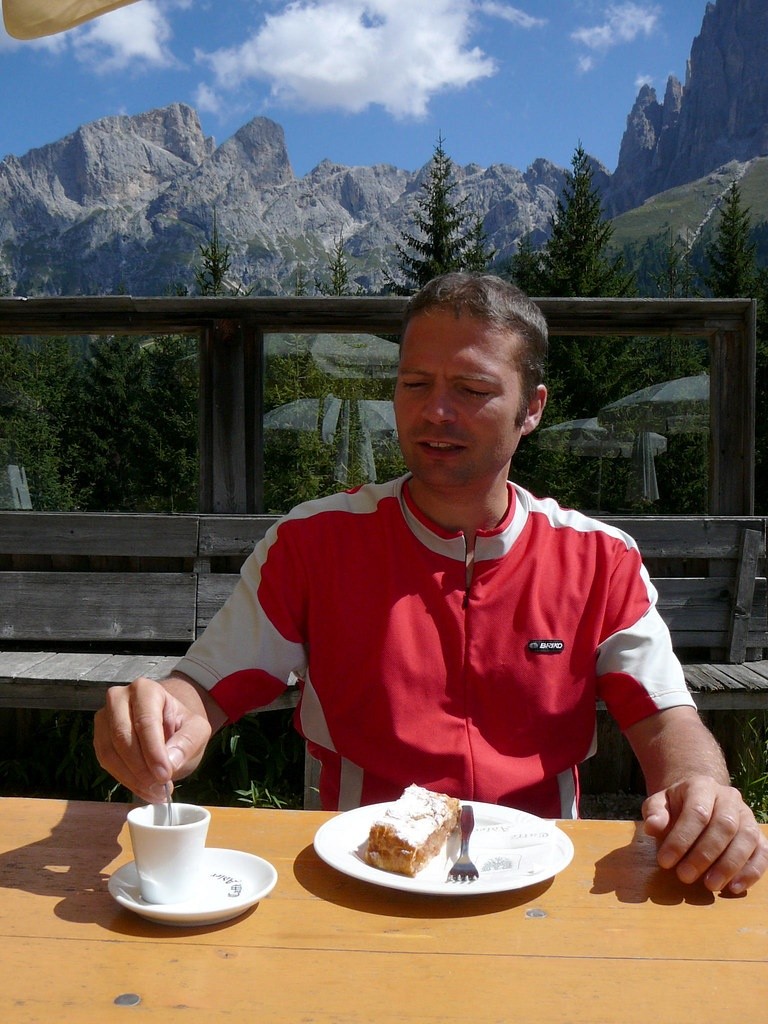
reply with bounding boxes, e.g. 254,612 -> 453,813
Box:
94,277 -> 768,895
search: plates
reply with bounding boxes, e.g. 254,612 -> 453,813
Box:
314,800 -> 574,896
108,848 -> 278,926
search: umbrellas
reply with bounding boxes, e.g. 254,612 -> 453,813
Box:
264,331 -> 400,490
537,370 -> 710,513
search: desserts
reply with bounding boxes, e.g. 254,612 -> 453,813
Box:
364,782 -> 463,877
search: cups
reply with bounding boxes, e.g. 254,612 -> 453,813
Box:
127,803 -> 211,904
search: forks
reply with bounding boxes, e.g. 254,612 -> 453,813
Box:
449,806 -> 479,882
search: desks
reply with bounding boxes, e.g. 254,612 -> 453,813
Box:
0,797 -> 768,1024
